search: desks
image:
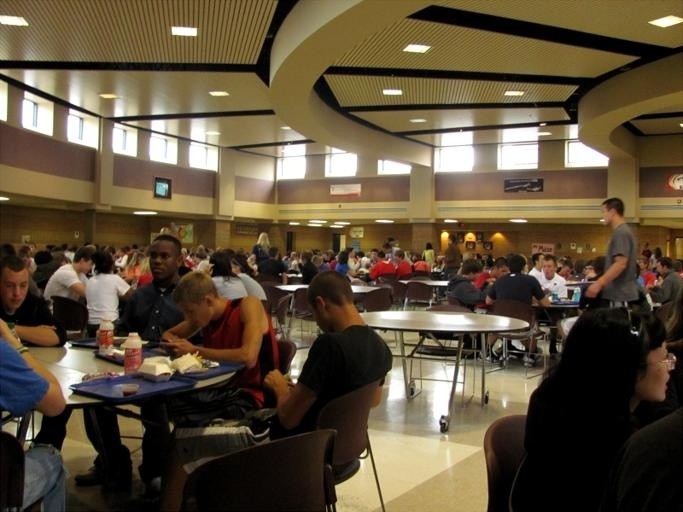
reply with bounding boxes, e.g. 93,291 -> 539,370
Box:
358,310 -> 529,431
275,284 -> 378,314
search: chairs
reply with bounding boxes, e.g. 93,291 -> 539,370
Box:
0,428 -> 23,512
263,286 -> 284,337
50,296 -> 89,339
403,282 -> 434,310
292,288 -> 320,343
153,429 -> 337,512
363,287 -> 393,335
416,306 -> 472,363
484,414 -> 538,512
484,301 -> 533,368
263,375 -> 394,512
535,300 -> 582,358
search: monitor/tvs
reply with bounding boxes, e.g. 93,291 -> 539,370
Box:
156,181 -> 169,197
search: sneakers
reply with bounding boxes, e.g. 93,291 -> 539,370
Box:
536,348 -> 543,362
492,338 -> 503,356
523,351 -> 536,367
478,351 -> 497,363
528,321 -> 546,339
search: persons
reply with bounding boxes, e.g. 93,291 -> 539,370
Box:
586,197 -> 639,309
203,251 -> 249,300
0,317 -> 68,512
162,271 -> 393,511
0,255 -> 73,451
448,258 -> 482,359
649,257 -> 683,307
283,243 -> 446,280
526,308 -> 671,512
487,253 -> 550,367
556,255 -> 611,340
227,247 -> 251,265
258,247 -> 298,315
639,242 -> 683,276
1,242 -> 76,263
248,232 -> 272,263
82,252 -> 135,339
161,270 -> 279,428
445,235 -> 462,268
182,243 -> 214,274
231,260 -> 267,301
474,258 -> 509,290
44,244 -> 96,314
75,236 -> 183,487
527,255 -> 559,357
97,243 -> 152,286
635,264 -> 651,311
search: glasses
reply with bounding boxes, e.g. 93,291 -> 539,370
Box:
649,353 -> 676,369
621,304 -> 643,337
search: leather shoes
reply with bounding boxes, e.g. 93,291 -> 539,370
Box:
75,465 -> 102,485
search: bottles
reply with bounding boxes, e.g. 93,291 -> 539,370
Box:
551,283 -> 558,302
98,318 -> 114,355
574,288 -> 580,302
124,332 -> 142,375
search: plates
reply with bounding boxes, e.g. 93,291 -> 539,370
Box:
94,347 -> 247,380
70,372 -> 198,404
68,336 -> 159,349
552,300 -> 580,305
564,280 -> 596,286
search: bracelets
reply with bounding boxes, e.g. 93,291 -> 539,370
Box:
18,346 -> 28,352
